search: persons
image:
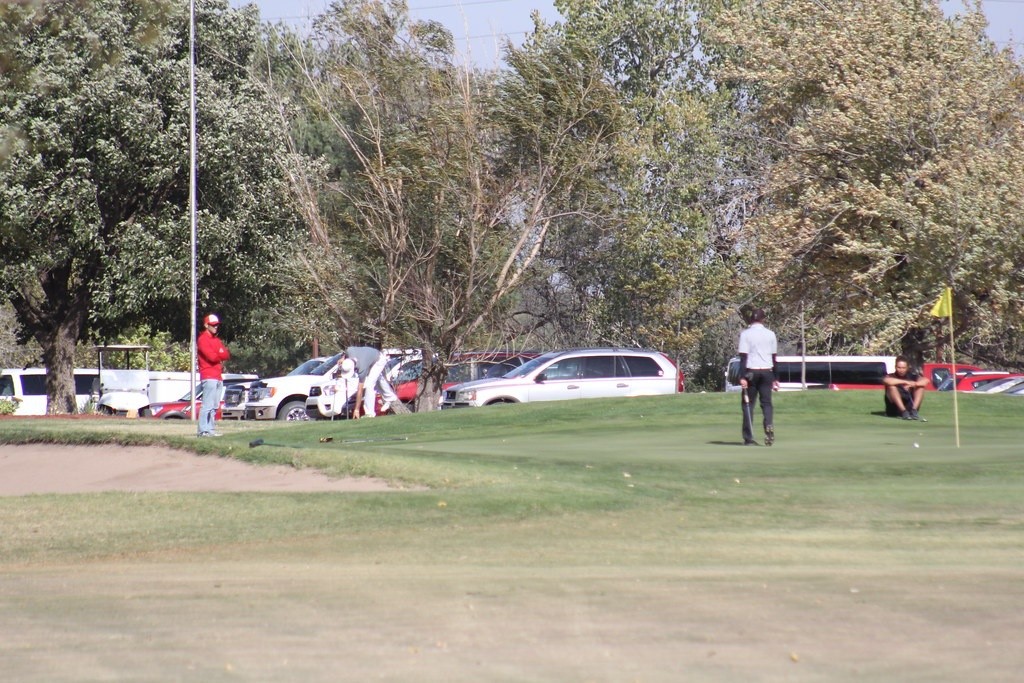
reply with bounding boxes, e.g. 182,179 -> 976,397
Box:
737,309 -> 779,447
341,347 -> 398,419
198,314 -> 230,436
883,356 -> 930,419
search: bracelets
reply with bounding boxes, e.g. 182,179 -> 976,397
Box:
355,410 -> 359,412
915,383 -> 917,386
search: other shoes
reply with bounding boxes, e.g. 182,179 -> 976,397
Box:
381,401 -> 391,412
911,411 -> 919,419
902,411 -> 912,420
765,425 -> 774,446
360,414 -> 375,418
744,440 -> 759,446
199,431 -> 222,438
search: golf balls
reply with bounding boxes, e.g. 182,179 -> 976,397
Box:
912,443 -> 919,448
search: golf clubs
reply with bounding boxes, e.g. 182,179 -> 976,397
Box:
744,388 -> 754,440
346,379 -> 349,420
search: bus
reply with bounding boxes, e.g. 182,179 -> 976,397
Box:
1,367 -> 257,415
725,354 -> 898,394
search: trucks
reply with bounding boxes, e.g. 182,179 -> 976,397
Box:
828,362 -> 984,391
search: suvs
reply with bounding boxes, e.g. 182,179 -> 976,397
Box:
937,371 -> 1024,397
221,347 -> 685,423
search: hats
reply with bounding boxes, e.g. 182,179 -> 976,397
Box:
752,310 -> 765,320
340,359 -> 354,378
205,315 -> 220,325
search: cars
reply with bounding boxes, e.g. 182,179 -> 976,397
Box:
143,378 -> 257,421
